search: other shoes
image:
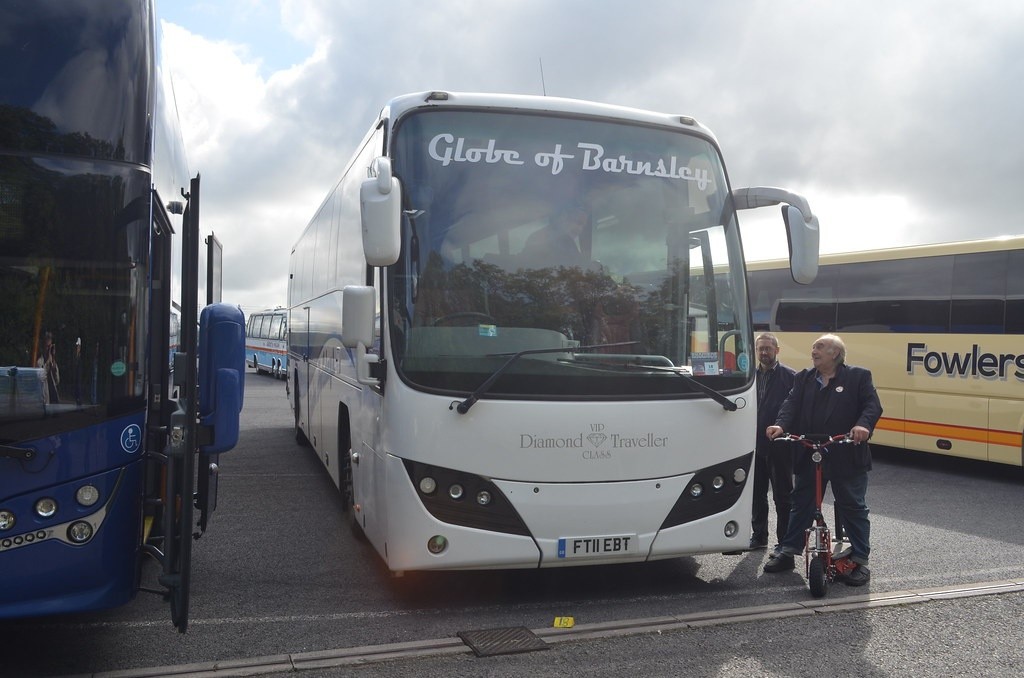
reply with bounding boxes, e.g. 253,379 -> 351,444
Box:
747,539 -> 768,549
769,544 -> 782,559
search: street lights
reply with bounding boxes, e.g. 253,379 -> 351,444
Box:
304,307 -> 309,356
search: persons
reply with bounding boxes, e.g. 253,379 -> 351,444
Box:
64,337 -> 86,408
763,334 -> 884,587
748,331 -> 798,558
34,328 -> 61,409
521,202 -> 589,263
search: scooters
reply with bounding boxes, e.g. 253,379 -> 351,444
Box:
768,430 -> 857,596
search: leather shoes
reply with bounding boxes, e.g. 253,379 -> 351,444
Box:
763,551 -> 795,573
845,566 -> 871,586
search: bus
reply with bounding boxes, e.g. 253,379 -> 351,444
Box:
168,299 -> 201,372
290,331 -> 354,364
1,0 -> 247,636
243,311 -> 290,380
283,87 -> 820,574
622,235 -> 1023,471
